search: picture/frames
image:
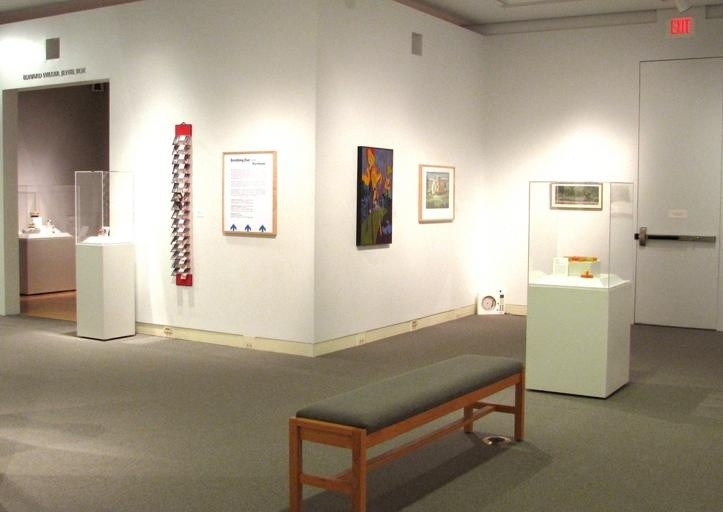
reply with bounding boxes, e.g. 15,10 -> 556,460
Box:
418,165 -> 455,222
223,151 -> 277,238
550,183 -> 602,210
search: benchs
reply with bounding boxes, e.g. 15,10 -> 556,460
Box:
290,354 -> 525,512
